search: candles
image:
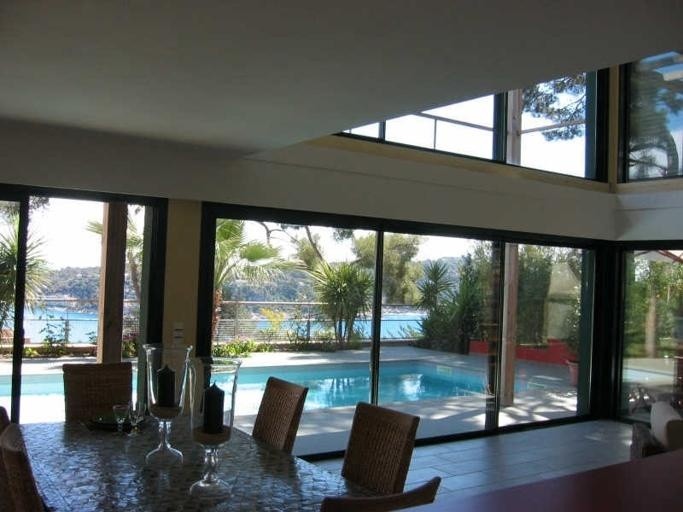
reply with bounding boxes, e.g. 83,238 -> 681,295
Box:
156,362 -> 175,407
202,380 -> 225,433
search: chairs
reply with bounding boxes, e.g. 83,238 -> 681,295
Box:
339,402 -> 420,495
62,362 -> 133,422
251,377 -> 309,457
1,407 -> 37,506
319,473 -> 441,512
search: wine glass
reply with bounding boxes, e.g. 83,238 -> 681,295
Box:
111,404 -> 127,435
185,358 -> 241,500
129,400 -> 141,434
143,344 -> 191,465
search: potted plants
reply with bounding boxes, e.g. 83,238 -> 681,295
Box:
557,288 -> 578,390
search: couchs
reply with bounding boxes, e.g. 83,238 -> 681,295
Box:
631,401 -> 682,462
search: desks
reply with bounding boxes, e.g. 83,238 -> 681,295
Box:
15,409 -> 376,512
